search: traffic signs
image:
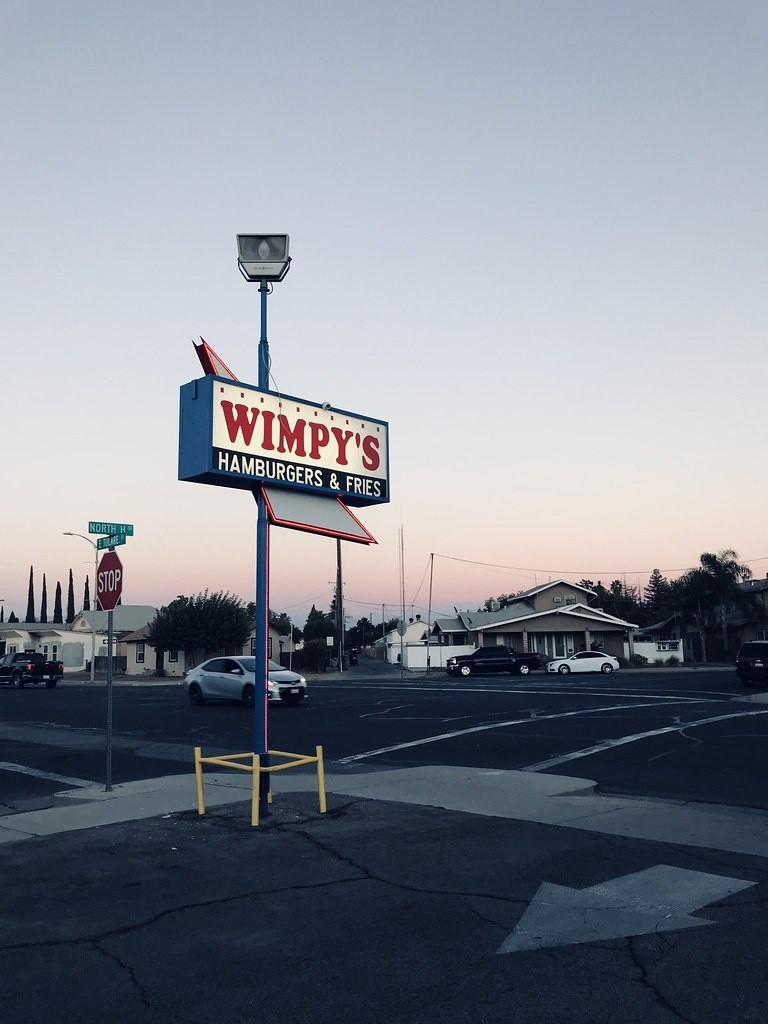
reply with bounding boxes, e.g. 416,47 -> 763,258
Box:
102,638 -> 118,644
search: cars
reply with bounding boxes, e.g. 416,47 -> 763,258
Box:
545,650 -> 620,675
182,655 -> 308,709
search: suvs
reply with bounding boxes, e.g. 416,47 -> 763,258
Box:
735,639 -> 768,687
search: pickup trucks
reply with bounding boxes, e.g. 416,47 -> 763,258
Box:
446,645 -> 544,677
0,652 -> 64,690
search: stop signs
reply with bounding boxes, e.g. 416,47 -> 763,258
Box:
96,550 -> 123,611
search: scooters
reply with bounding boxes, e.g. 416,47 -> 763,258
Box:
350,652 -> 358,667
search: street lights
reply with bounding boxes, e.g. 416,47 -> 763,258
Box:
63,531 -> 99,682
232,234 -> 292,820
